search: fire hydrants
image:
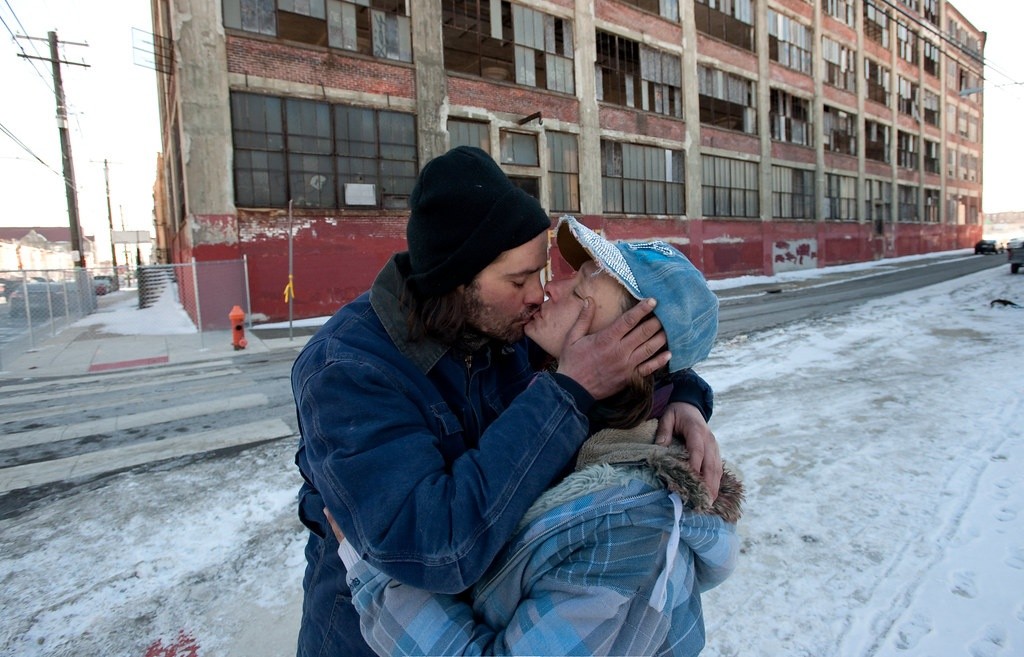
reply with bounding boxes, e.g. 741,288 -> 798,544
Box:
229,305 -> 247,351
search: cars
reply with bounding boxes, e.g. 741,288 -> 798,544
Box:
94,280 -> 106,295
0,275 -> 55,297
103,280 -> 113,293
61,280 -> 78,310
9,282 -> 65,317
975,240 -> 1004,255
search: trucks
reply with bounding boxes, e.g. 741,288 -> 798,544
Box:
1007,248 -> 1024,273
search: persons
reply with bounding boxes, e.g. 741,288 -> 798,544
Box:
292,143 -> 726,657
323,214 -> 747,656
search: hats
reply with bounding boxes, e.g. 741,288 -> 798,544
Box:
555,212 -> 719,374
407,145 -> 552,300
